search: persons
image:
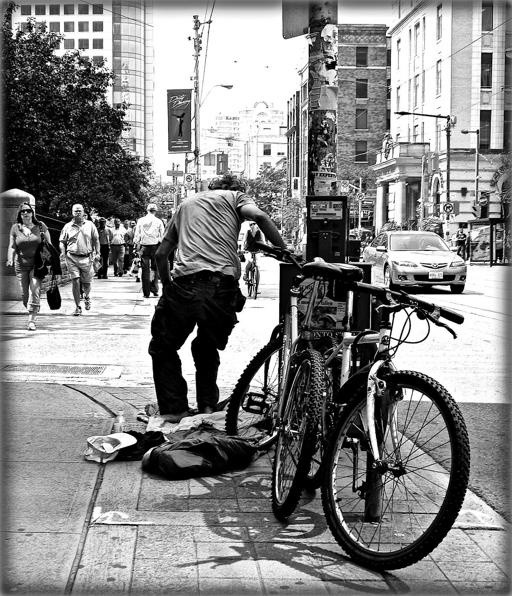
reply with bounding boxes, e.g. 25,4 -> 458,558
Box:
496,237 -> 503,262
456,229 -> 466,262
6,202 -> 62,330
148,174 -> 295,423
59,203 -> 267,316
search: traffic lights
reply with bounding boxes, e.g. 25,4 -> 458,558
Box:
472,204 -> 481,217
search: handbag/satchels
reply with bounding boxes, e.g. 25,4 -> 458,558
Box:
18,254 -> 35,269
47,274 -> 61,310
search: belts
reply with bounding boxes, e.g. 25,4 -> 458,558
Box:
69,252 -> 92,257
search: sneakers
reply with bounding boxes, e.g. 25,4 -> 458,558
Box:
85,304 -> 90,310
27,320 -> 37,331
74,307 -> 81,316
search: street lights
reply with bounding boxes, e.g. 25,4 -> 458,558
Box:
460,127 -> 482,218
393,109 -> 454,220
193,82 -> 233,190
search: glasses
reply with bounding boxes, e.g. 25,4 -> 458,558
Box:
19,209 -> 32,213
